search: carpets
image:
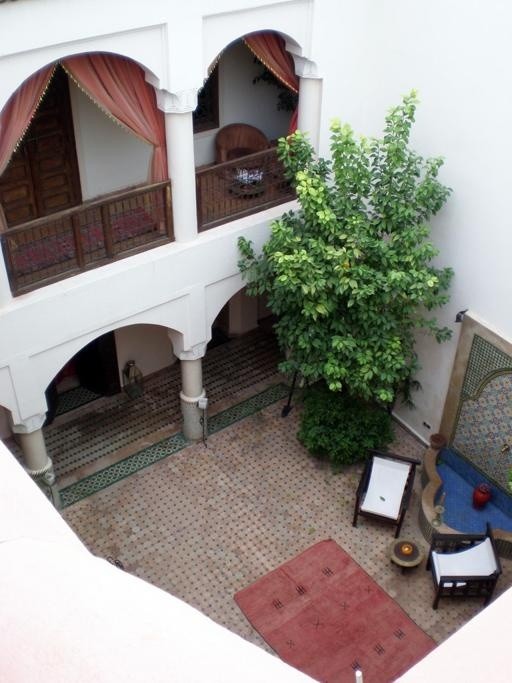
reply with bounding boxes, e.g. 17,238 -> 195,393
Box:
232,537 -> 439,682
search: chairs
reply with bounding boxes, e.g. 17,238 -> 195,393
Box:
214,123 -> 269,182
427,522 -> 502,610
353,447 -> 421,538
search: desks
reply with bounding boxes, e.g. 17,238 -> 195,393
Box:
228,180 -> 266,200
